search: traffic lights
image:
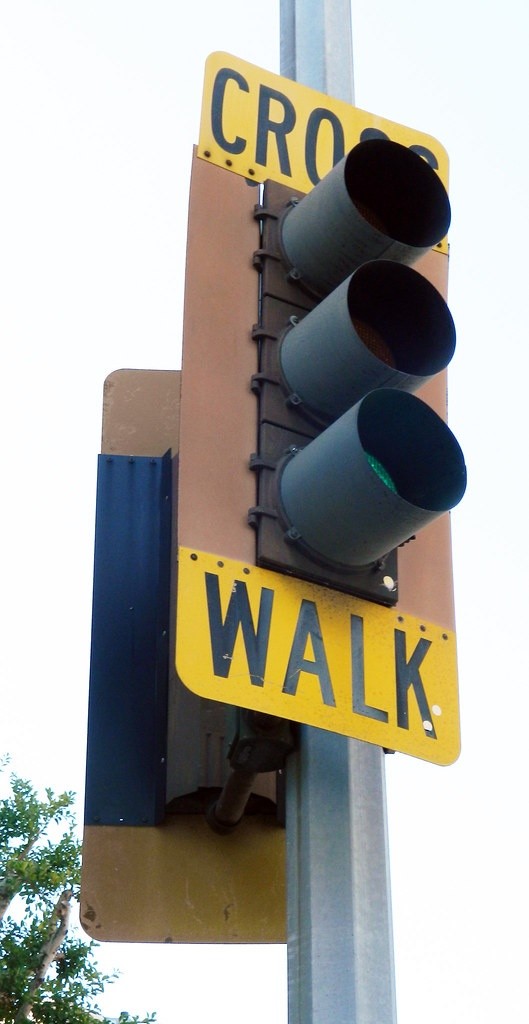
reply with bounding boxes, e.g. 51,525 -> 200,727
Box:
247,139 -> 467,608
